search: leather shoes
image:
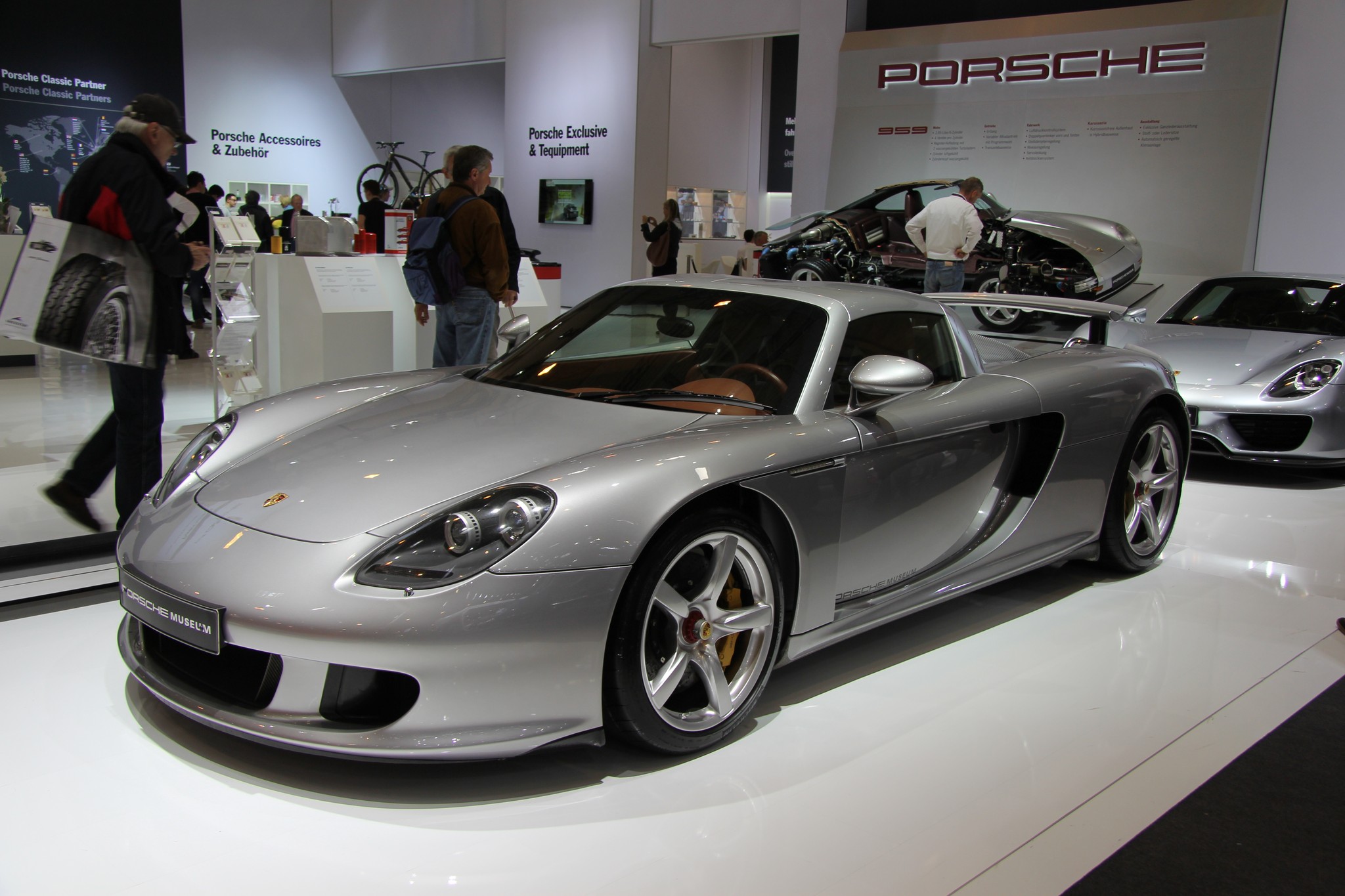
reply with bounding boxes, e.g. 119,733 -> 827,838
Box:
45,479 -> 101,531
178,348 -> 199,360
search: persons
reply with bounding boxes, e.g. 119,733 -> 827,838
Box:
413,145 -> 522,363
279,194 -> 314,253
399,186 -> 426,218
357,179 -> 395,254
271,194 -> 295,223
640,198 -> 683,277
218,193 -> 237,218
377,183 -> 390,203
905,176 -> 984,293
0,198 -> 15,233
741,231 -> 770,278
173,171 -> 225,328
415,144 -> 511,369
736,229 -> 757,278
206,184 -> 224,203
237,189 -> 275,253
160,165 -> 202,360
43,92 -> 215,533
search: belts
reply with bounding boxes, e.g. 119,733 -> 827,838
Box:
927,258 -> 964,264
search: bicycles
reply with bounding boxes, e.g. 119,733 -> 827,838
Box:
356,142 -> 448,210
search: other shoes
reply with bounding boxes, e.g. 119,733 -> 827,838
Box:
191,319 -> 204,329
185,319 -> 194,325
184,286 -> 191,299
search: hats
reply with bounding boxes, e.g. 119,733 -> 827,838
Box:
123,93 -> 196,144
379,184 -> 394,191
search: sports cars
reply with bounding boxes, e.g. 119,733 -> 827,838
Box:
116,272 -> 1191,764
1063,269 -> 1345,468
761,178 -> 1140,332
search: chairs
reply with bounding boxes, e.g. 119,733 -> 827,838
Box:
904,189 -> 924,223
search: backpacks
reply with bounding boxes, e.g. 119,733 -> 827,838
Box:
402,186 -> 482,306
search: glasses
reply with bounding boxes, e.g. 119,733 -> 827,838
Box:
229,199 -> 236,201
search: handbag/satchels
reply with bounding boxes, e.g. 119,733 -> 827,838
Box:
646,221 -> 670,267
0,215 -> 155,367
